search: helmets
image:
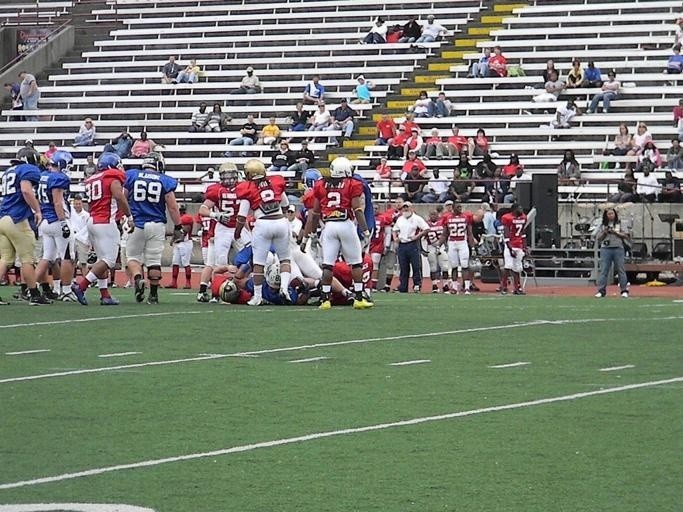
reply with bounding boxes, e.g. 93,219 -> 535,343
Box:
219,162 -> 237,174
219,281 -> 240,302
142,152 -> 165,174
50,150 -> 74,172
301,168 -> 323,189
330,157 -> 353,178
17,148 -> 40,165
266,263 -> 283,289
244,159 -> 266,180
97,152 -> 123,171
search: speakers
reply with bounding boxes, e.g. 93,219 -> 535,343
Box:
515,182 -> 531,246
532,173 -> 559,247
632,243 -> 647,257
652,242 -> 672,259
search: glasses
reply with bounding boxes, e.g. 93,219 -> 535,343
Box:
287,210 -> 294,213
281,144 -> 287,145
86,121 -> 92,123
638,126 -> 645,128
318,105 -> 324,107
608,74 -> 614,76
50,145 -> 55,147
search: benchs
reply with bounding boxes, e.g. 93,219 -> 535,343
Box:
0,0 -> 683,203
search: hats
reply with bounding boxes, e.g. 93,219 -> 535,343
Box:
399,126 -> 406,129
25,139 -> 34,144
341,98 -> 347,102
288,205 -> 296,211
402,201 -> 411,206
515,164 -> 523,169
357,75 -> 364,81
481,202 -> 491,211
445,200 -> 453,206
509,153 -> 518,158
411,128 -> 418,131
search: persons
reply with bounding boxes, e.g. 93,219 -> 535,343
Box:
0,13 -> 683,310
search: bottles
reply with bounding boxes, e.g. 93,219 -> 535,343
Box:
602,161 -> 608,169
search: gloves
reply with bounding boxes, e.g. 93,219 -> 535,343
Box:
215,212 -> 230,224
61,222 -> 70,238
173,225 -> 186,242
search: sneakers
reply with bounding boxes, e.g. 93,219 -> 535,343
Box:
501,289 -> 507,294
0,276 -> 160,305
595,292 -> 606,297
312,297 -> 332,309
279,289 -> 292,305
353,298 -> 374,309
165,282 -> 177,288
372,280 -> 480,295
622,292 -> 628,297
512,289 -> 526,295
247,298 -> 265,306
183,283 -> 191,289
197,293 -> 209,302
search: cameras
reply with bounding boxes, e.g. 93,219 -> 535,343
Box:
608,226 -> 613,233
